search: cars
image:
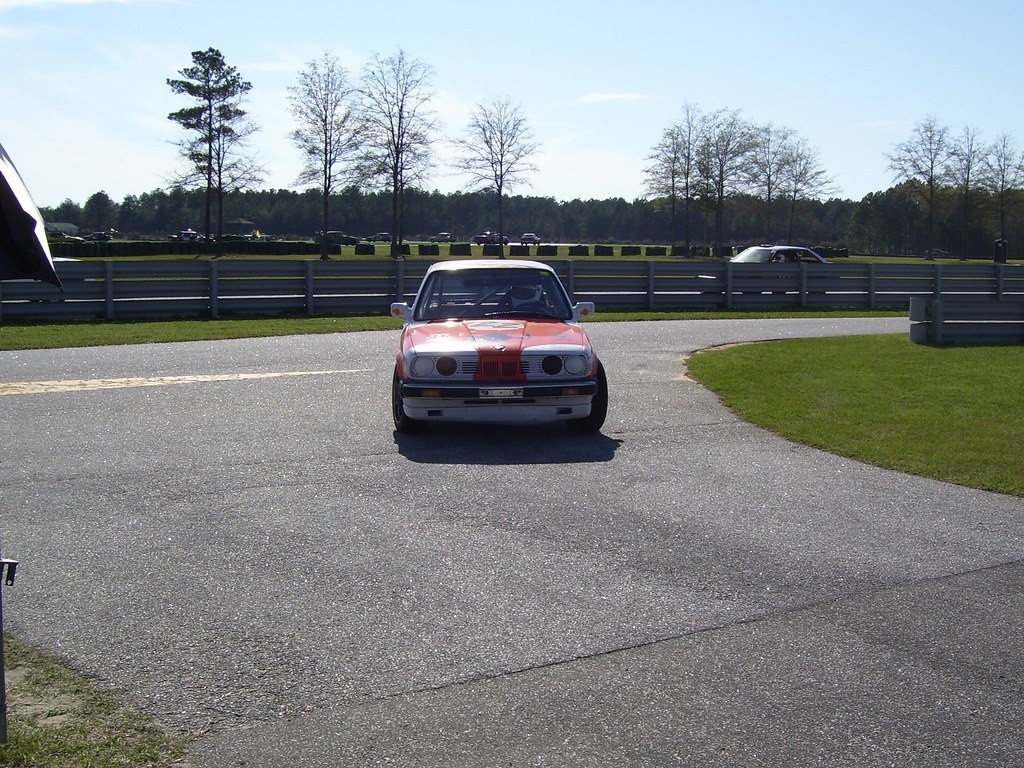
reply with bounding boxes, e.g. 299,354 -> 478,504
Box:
699,244 -> 835,294
314,230 -> 360,246
520,233 -> 540,245
923,249 -> 950,259
391,259 -> 608,434
366,232 -> 392,242
429,233 -> 451,243
475,232 -> 510,246
81,232 -> 110,241
168,230 -> 214,242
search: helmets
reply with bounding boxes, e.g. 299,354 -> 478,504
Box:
509,283 -> 542,307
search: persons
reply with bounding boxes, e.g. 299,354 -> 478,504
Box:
509,281 -> 549,313
771,253 -> 785,264
